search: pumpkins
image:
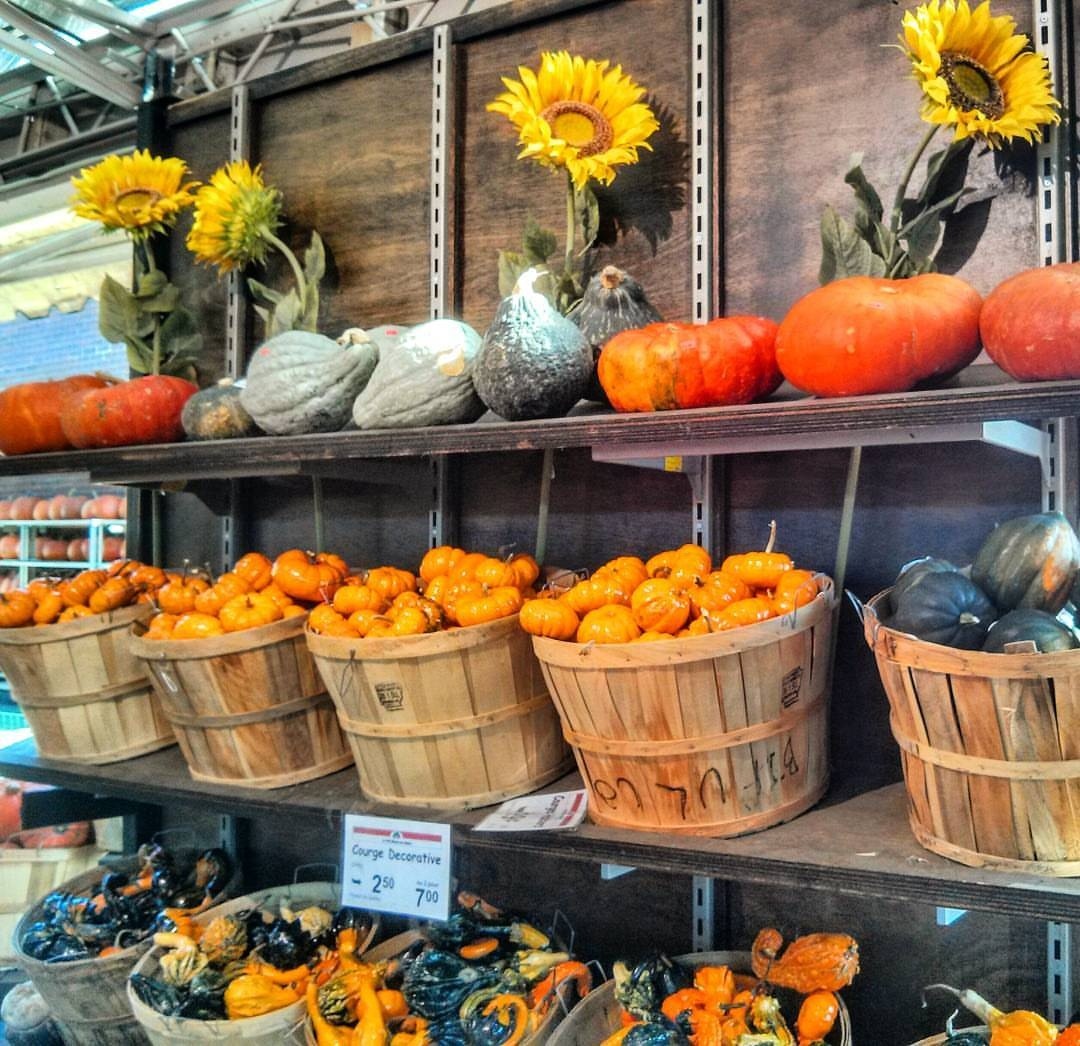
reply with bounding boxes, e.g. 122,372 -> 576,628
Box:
0,780 -> 1079,1046
0,490 -> 824,645
884,507 -> 1080,654
0,260 -> 1080,455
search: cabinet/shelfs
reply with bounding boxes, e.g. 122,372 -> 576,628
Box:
0,361 -> 1079,925
